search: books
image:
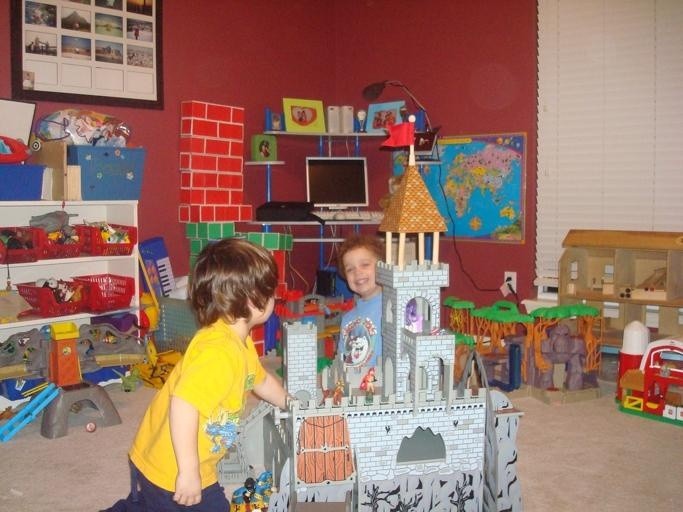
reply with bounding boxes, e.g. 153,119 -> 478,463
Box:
414,132 -> 438,157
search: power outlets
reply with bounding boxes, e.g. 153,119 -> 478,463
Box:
504,271 -> 516,294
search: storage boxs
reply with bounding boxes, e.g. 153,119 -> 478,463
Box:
68,145 -> 145,200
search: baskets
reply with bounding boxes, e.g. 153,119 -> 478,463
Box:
73,274 -> 136,314
14,280 -> 86,316
1,222 -> 137,263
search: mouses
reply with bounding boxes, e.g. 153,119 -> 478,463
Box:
334,212 -> 346,221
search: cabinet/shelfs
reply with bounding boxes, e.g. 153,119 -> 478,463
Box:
243,130 -> 389,272
0,200 -> 140,416
558,229 -> 683,357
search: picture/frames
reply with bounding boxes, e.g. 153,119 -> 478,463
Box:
365,101 -> 405,133
391,131 -> 528,245
10,0 -> 165,111
282,97 -> 326,134
250,135 -> 278,161
271,113 -> 285,130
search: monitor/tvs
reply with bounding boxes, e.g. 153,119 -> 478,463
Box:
306,156 -> 369,211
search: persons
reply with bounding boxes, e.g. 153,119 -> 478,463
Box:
133,25 -> 139,40
336,233 -> 386,370
27,38 -> 50,54
320,386 -> 331,406
332,379 -> 345,406
373,111 -> 394,128
232,471 -> 278,512
93,238 -> 291,511
298,110 -> 306,122
361,366 -> 377,399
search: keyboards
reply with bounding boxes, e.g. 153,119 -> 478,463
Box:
310,211 -> 372,221
